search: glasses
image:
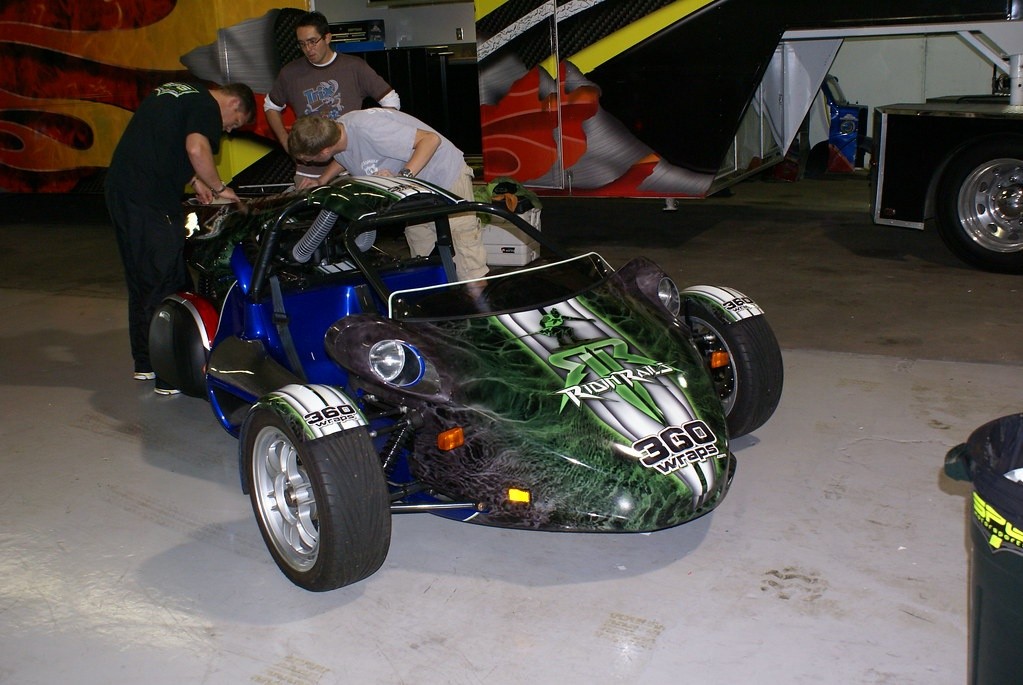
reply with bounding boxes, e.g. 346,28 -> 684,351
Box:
294,36 -> 323,48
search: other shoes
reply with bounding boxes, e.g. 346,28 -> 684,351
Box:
134,361 -> 156,379
154,376 -> 182,395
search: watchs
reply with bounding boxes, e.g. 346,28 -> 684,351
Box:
399,168 -> 413,178
210,180 -> 226,193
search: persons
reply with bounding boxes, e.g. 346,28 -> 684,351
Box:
262,12 -> 400,188
104,81 -> 257,395
288,108 -> 490,281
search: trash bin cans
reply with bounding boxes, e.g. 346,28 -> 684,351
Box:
942,410 -> 1023,685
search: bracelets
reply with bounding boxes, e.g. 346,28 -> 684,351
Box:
191,178 -> 199,187
